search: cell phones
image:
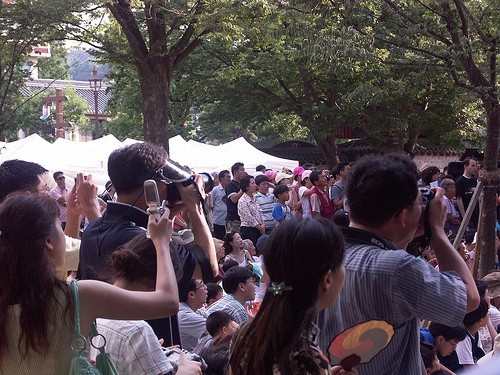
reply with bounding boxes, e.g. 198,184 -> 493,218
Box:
144,180 -> 166,240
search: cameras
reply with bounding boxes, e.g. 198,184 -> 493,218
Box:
167,172 -> 213,203
415,186 -> 434,238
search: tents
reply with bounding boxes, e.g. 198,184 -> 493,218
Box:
1,133 -> 299,173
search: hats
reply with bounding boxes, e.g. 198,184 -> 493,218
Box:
275,173 -> 293,184
294,167 -> 305,177
302,170 -> 312,182
107,183 -> 112,192
178,229 -> 195,245
213,237 -> 224,252
111,158 -> 193,190
265,170 -> 277,181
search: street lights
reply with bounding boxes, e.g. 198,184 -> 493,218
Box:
88,64 -> 102,138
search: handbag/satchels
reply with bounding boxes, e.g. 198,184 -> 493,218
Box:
69,279 -> 118,375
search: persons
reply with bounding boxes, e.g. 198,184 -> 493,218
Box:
0,141 -> 500,375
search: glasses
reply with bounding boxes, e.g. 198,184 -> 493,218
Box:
56,177 -> 65,180
449,341 -> 458,349
395,197 -> 429,217
